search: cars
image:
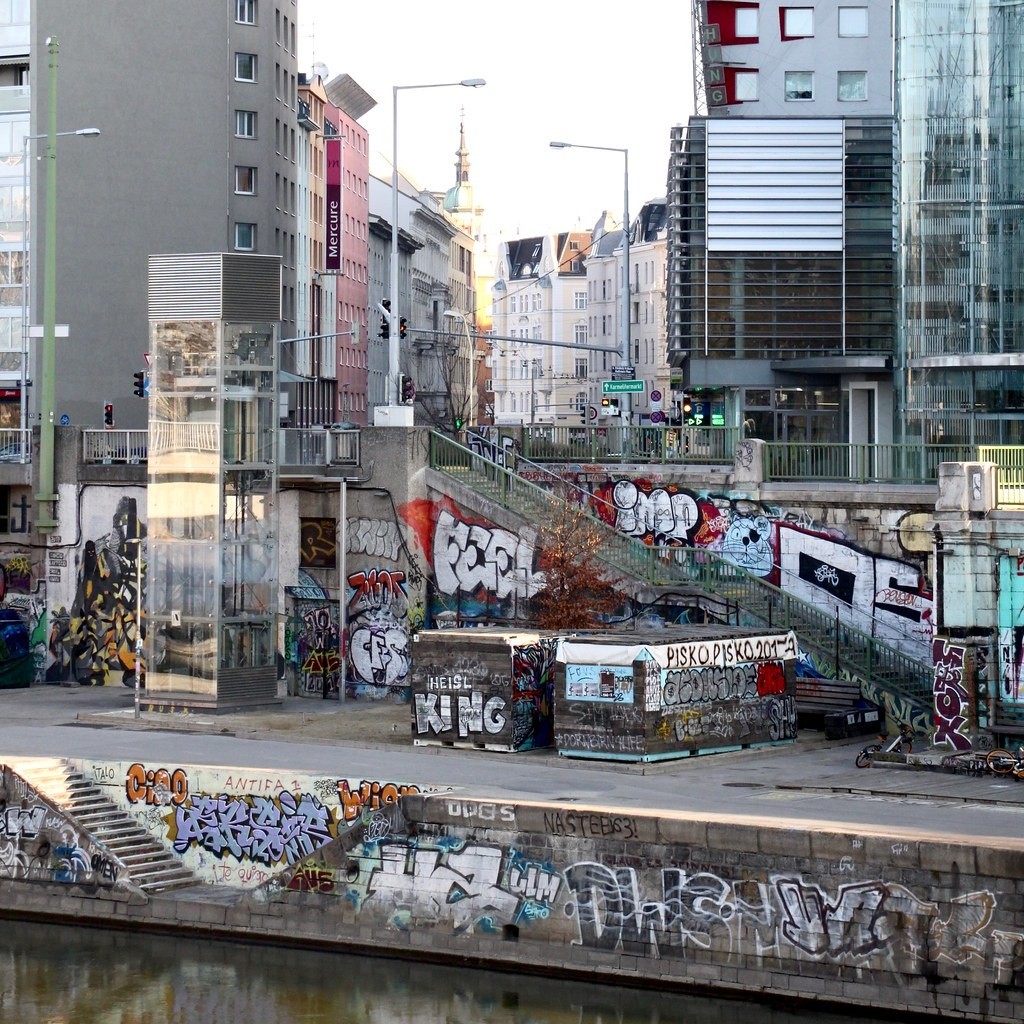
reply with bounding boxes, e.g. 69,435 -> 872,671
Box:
0,442 -> 32,465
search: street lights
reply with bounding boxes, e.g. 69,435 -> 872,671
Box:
388,75 -> 487,407
548,140 -> 633,367
444,309 -> 475,426
17,125 -> 101,464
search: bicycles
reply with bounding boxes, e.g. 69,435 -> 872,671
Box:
985,745 -> 1024,782
855,716 -> 918,768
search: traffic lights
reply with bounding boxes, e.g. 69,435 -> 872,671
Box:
382,299 -> 391,324
454,415 -> 461,429
132,370 -> 145,398
381,325 -> 389,339
105,405 -> 114,425
403,375 -> 414,404
601,398 -> 609,406
684,397 -> 692,415
400,317 -> 407,339
